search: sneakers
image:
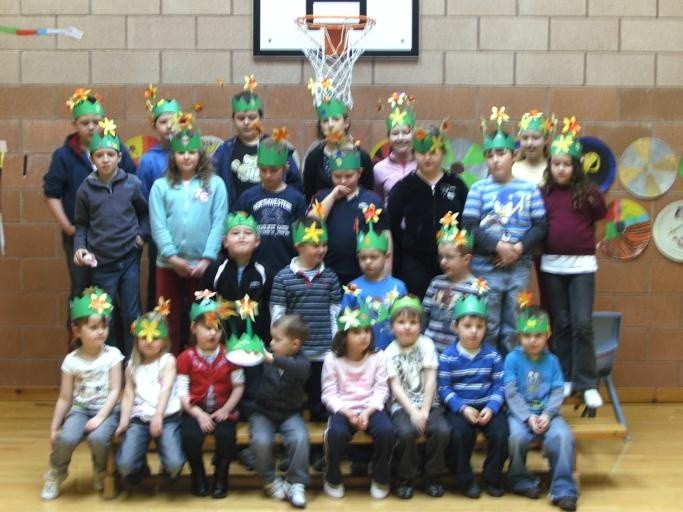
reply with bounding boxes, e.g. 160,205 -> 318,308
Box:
41,471 -> 67,501
324,483 -> 344,498
487,483 -> 503,497
525,489 -> 538,498
93,471 -> 104,492
558,497 -> 575,511
584,389 -> 603,409
463,484 -> 480,499
370,481 -> 389,498
283,482 -> 305,507
265,481 -> 285,498
563,382 -> 574,399
393,484 -> 413,499
426,483 -> 444,498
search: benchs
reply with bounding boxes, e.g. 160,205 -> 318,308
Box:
104,417 -> 627,499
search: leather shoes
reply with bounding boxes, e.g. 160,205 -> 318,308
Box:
193,479 -> 209,496
213,477 -> 227,498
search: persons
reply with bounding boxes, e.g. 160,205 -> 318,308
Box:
437,295 -> 510,498
504,306 -> 575,511
271,217 -> 341,363
212,91 -> 302,211
114,314 -> 185,489
421,226 -> 484,365
334,228 -> 408,350
142,99 -> 190,317
304,151 -> 384,294
248,312 -> 310,507
44,88 -> 137,359
540,134 -> 606,409
461,130 -> 549,357
230,142 -> 307,289
42,290 -> 121,500
146,127 -> 227,354
171,299 -> 245,498
375,107 -> 437,207
196,215 -> 275,346
71,134 -> 149,365
378,132 -> 469,298
508,114 -> 553,185
321,311 -> 395,499
384,295 -> 450,499
303,97 -> 373,204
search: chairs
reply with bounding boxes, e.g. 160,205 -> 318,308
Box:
574,312 -> 632,440
